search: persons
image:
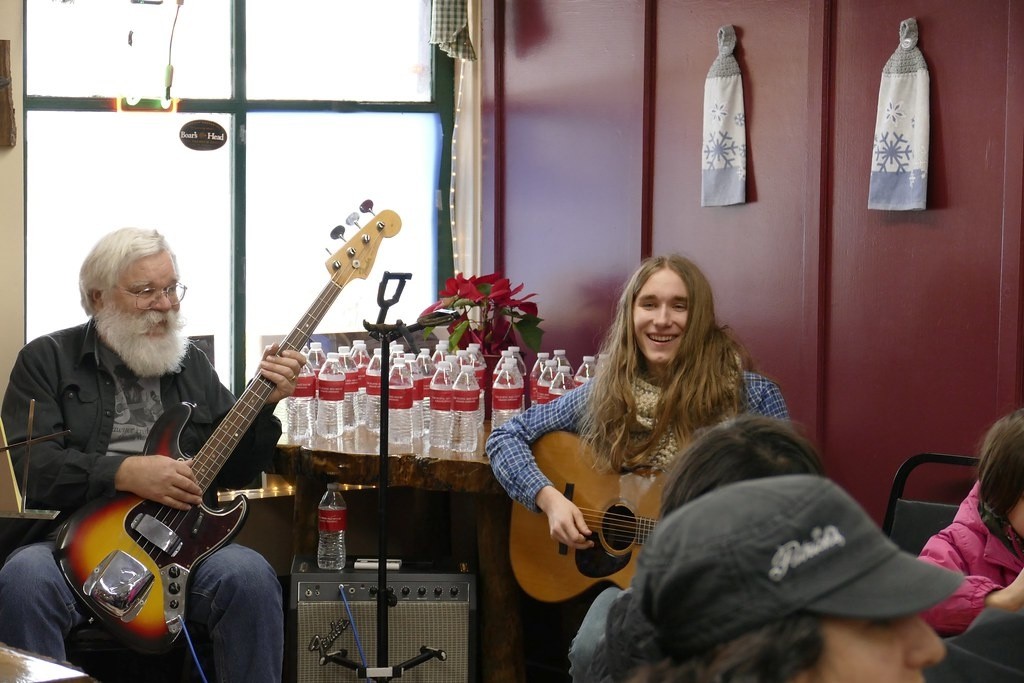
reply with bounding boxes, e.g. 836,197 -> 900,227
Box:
0,227 -> 307,683
485,254 -> 1023,683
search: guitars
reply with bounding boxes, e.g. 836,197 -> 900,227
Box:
51,200 -> 403,656
509,431 -> 672,604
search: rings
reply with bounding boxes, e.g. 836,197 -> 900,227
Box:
288,373 -> 297,383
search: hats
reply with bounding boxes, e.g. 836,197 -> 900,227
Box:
634,475 -> 963,663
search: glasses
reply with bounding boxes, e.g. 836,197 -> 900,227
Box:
112,283 -> 189,310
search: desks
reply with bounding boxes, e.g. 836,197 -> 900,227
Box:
275,419 -> 529,682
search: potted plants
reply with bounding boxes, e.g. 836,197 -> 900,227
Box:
416,272 -> 549,419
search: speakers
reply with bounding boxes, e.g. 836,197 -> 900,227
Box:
288,550 -> 480,682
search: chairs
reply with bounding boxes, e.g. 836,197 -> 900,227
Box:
882,451 -> 983,555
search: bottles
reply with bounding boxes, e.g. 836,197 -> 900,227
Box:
318,481 -> 348,571
280,340 -> 614,456
